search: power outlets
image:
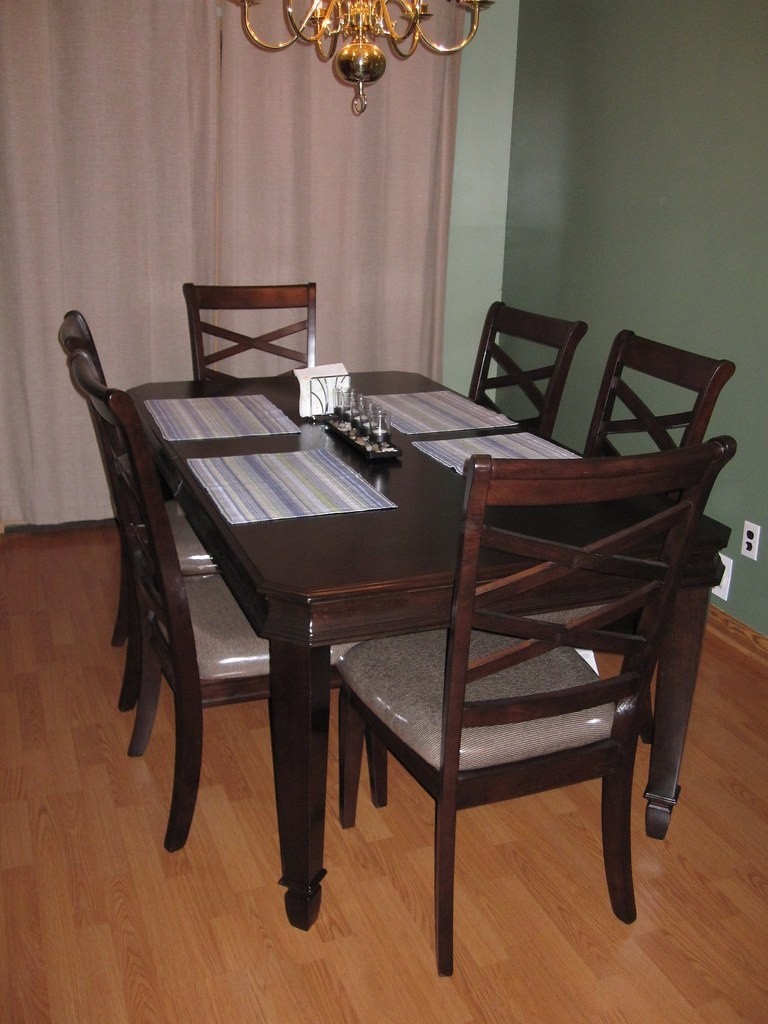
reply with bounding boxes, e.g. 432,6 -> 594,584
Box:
742,520 -> 762,561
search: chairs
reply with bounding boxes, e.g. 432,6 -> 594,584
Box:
55,278 -> 738,976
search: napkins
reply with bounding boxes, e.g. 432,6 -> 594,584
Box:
292,363 -> 354,418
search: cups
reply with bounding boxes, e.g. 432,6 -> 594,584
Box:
340,392 -> 364,422
350,398 -> 373,430
368,410 -> 391,443
359,404 -> 382,442
332,388 -> 354,415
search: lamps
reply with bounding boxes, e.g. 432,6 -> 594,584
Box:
233,0 -> 493,116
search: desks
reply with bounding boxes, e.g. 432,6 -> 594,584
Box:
129,369 -> 733,931
